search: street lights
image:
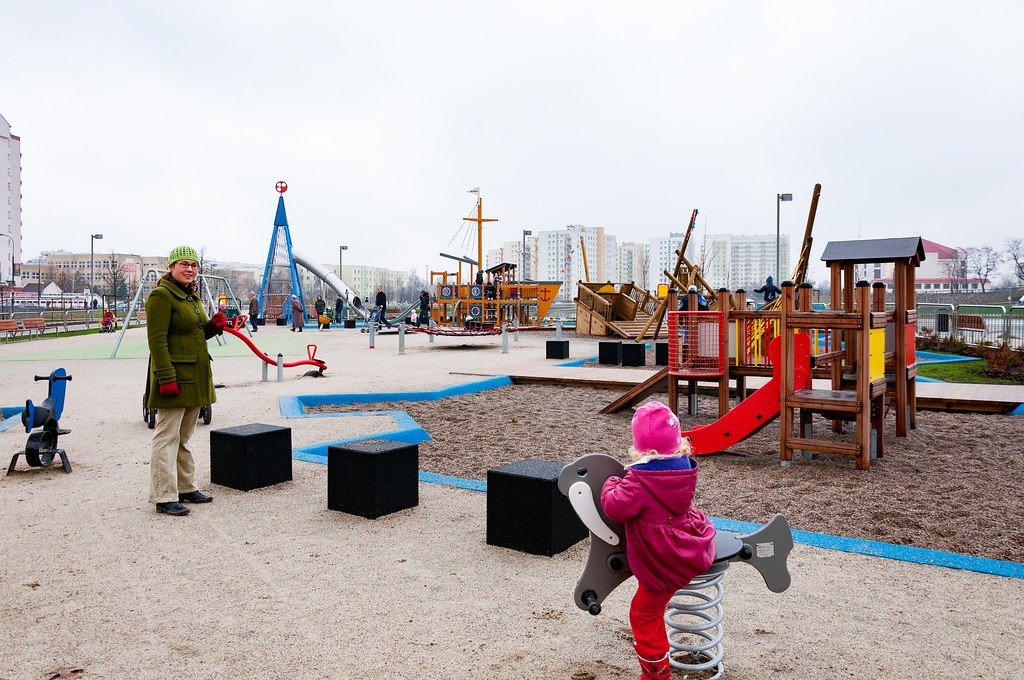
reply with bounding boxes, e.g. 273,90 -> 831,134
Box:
38,253 -> 48,309
0,233 -> 14,313
340,245 -> 348,280
777,193 -> 793,286
522,230 -> 532,280
91,234 -> 103,310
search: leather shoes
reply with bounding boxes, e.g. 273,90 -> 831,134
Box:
179,491 -> 214,502
156,501 -> 190,515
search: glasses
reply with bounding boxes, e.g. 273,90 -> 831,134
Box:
176,260 -> 200,270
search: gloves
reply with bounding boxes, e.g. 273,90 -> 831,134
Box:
160,382 -> 180,396
211,312 -> 227,327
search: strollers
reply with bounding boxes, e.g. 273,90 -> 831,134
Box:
360,306 -> 383,333
99,311 -> 115,333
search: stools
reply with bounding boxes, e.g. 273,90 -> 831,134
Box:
328,438 -> 420,520
546,340 -> 569,359
599,341 -> 622,365
318,321 -> 329,329
344,320 -> 356,328
277,318 -> 287,325
210,423 -> 293,493
486,458 -> 590,558
622,343 -> 646,367
420,317 -> 431,327
656,343 -> 668,366
257,318 -> 265,325
405,317 -> 419,327
683,344 -> 689,363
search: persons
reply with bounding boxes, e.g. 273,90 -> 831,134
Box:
678,284 -> 710,346
493,271 -> 503,287
751,276 -> 782,306
315,296 -> 325,329
290,295 -> 304,333
334,294 -> 343,323
475,270 -> 484,285
374,287 -> 391,329
415,291 -> 431,328
486,283 -> 496,300
601,401 -> 716,680
248,291 -> 260,332
143,245 -> 226,515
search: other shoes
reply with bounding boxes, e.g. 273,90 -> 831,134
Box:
291,329 -> 295,331
388,323 -> 391,329
251,330 -> 257,332
298,330 -> 302,332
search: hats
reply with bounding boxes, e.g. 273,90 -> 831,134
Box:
168,246 -> 201,267
292,295 -> 296,298
689,285 -> 697,292
631,401 -> 681,455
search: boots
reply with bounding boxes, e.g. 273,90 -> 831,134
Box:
636,653 -> 671,680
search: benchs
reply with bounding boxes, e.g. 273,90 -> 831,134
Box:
137,311 -> 147,325
465,322 -> 494,332
0,319 -> 32,344
21,318 -> 58,340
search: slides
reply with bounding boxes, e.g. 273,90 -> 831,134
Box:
680,330 -> 812,457
388,294 -> 436,324
291,251 -> 372,321
598,361 -> 691,413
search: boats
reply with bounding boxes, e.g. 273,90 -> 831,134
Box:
426,196 -> 563,328
